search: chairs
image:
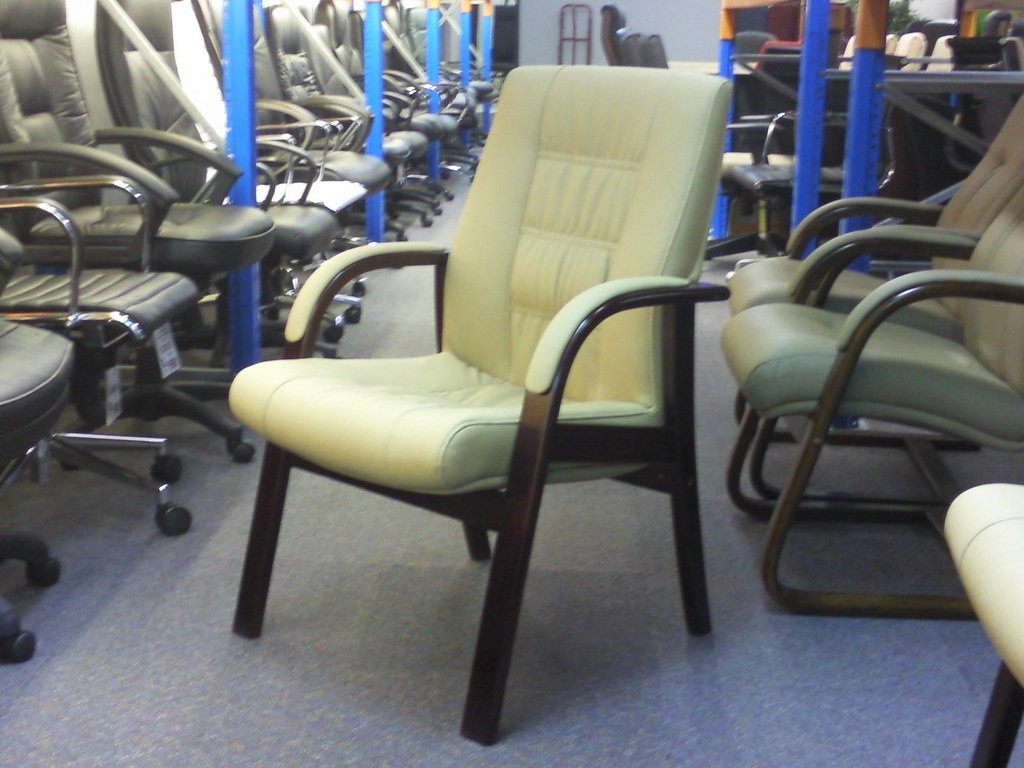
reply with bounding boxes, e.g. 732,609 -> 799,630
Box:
941,481 -> 1024,768
97,0 -> 339,393
228,65 -> 735,748
0,196 -> 193,664
727,94 -> 1024,504
702,99 -> 894,263
719,184 -> 1024,617
841,10 -> 1024,97
0,0 -> 276,463
0,174 -> 191,536
192,0 -> 394,297
599,5 -> 668,66
702,70 -> 895,281
266,0 -> 497,241
